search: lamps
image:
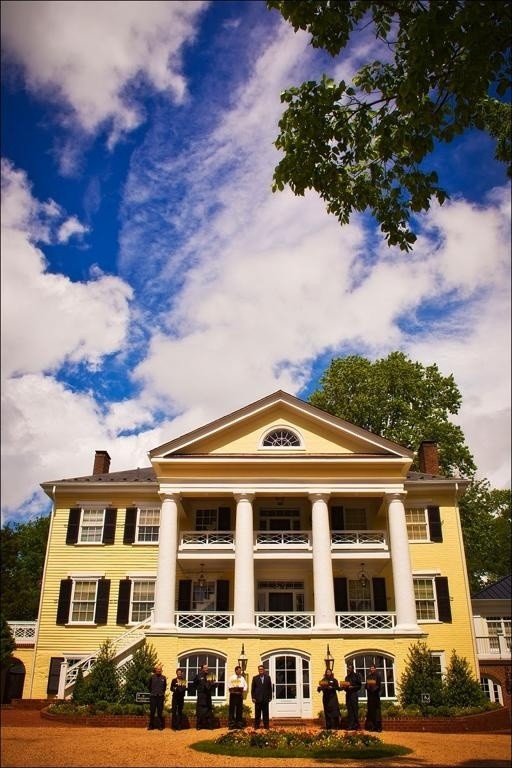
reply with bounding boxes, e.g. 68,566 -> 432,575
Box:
197,563 -> 206,588
357,563 -> 370,587
237,643 -> 249,673
324,643 -> 335,671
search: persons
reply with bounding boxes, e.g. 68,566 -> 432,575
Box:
250,665 -> 273,730
317,668 -> 341,730
226,666 -> 248,720
344,664 -> 363,730
193,663 -> 216,730
364,664 -> 384,732
146,665 -> 166,730
170,668 -> 187,730
